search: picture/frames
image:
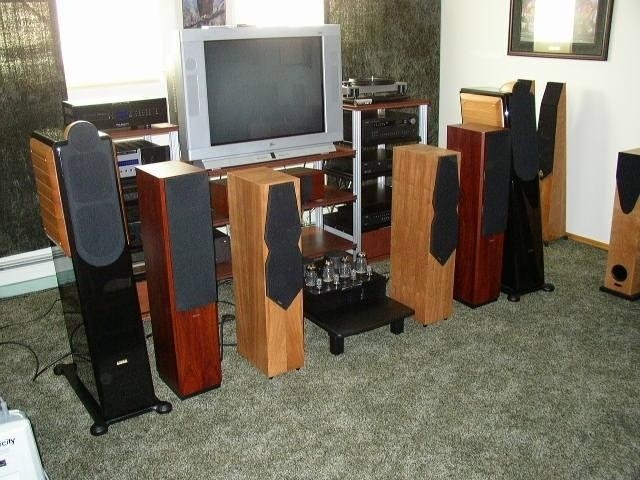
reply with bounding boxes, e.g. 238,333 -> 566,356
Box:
507,0 -> 613,61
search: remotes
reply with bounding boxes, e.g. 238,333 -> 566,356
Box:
372,95 -> 408,102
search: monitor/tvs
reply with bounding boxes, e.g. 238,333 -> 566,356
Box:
174,24 -> 344,171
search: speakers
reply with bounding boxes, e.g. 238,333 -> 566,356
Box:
537,81 -> 568,247
446,122 -> 511,309
511,79 -> 535,95
390,144 -> 462,327
28,120 -> 155,408
599,147 -> 640,301
134,159 -> 222,401
459,85 -> 555,301
226,164 -> 305,378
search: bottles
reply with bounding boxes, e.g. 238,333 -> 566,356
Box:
304,263 -> 317,287
321,257 -> 334,283
355,251 -> 368,275
339,255 -> 352,278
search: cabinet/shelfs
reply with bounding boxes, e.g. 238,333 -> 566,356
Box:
317,97 -> 436,263
206,145 -> 357,284
303,271 -> 416,356
102,122 -> 183,324
